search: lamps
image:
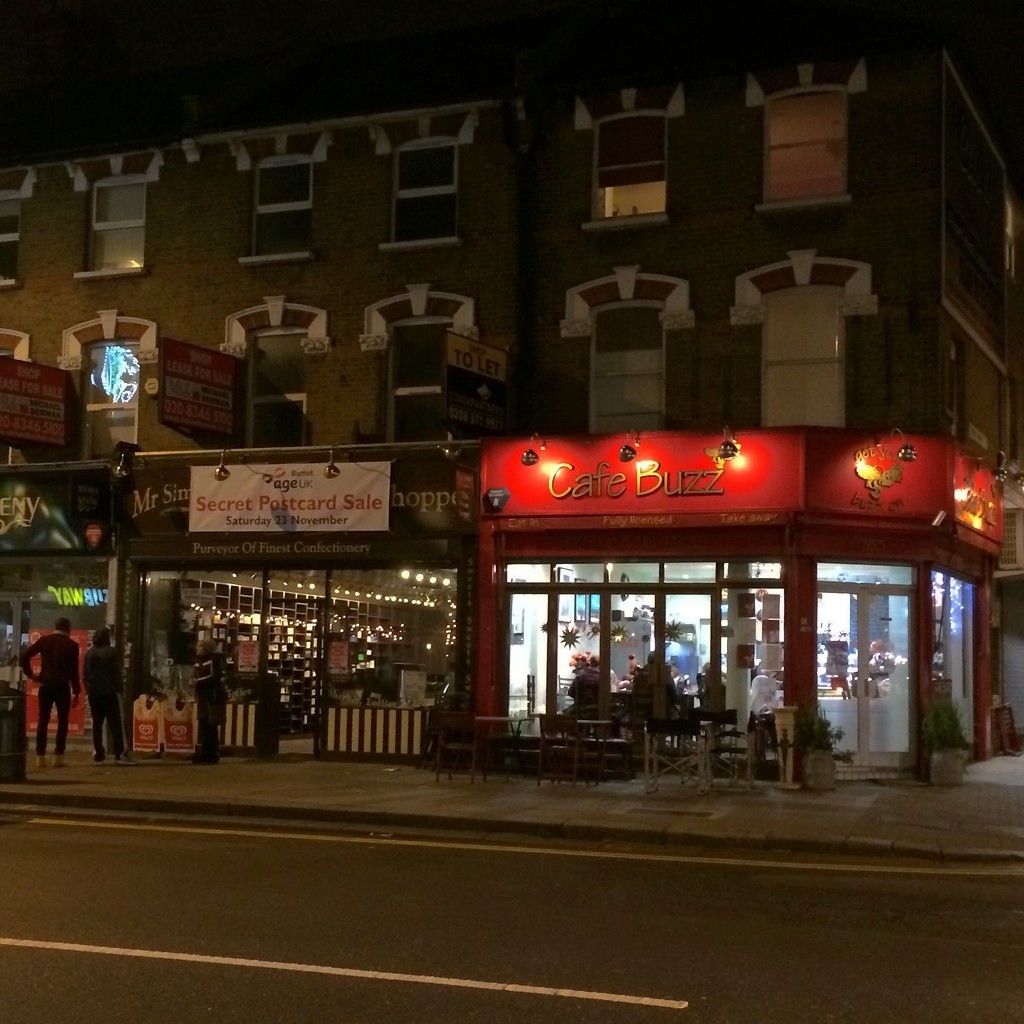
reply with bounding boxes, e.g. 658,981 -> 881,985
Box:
111,426 -> 1024,487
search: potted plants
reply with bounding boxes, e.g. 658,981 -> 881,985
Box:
793,699 -> 859,790
920,678 -> 977,786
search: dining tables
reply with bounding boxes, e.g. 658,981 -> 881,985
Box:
609,690 -> 700,750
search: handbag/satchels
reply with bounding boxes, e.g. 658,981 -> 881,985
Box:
207,701 -> 226,725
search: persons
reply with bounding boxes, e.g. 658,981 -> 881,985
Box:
19,618 -> 80,767
192,639 -> 225,765
83,627 -> 126,761
869,640 -> 895,672
569,651 -> 725,718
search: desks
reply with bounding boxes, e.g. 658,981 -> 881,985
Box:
474,716 -> 534,784
575,720 -> 614,787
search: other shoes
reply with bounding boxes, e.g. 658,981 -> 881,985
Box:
35,755 -> 50,768
189,752 -> 219,765
112,751 -> 139,765
92,755 -> 105,765
51,754 -> 68,768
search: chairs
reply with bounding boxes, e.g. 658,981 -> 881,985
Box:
536,714 -> 591,788
643,708 -> 749,796
526,675 -> 575,719
433,709 -> 488,785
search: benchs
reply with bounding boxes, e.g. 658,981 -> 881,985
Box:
491,733 -> 631,780
